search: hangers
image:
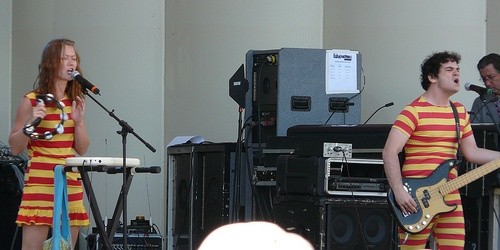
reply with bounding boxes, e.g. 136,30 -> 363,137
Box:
0,143 -> 30,167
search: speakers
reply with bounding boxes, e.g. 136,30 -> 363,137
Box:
272,194 -> 398,250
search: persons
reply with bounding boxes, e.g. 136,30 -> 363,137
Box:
8,38 -> 90,250
470,52 -> 500,123
382,50 -> 500,250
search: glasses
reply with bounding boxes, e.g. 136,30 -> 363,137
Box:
478,71 -> 497,86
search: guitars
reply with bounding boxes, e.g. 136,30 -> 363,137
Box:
388,157 -> 500,235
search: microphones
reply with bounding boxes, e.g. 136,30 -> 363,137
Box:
364,102 -> 394,124
464,83 -> 493,96
471,96 -> 499,123
71,71 -> 100,95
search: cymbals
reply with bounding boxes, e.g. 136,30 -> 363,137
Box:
23,93 -> 69,140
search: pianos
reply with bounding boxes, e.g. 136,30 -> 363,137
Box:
287,121 -> 500,135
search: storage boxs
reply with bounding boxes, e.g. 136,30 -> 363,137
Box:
85,231 -> 164,250
